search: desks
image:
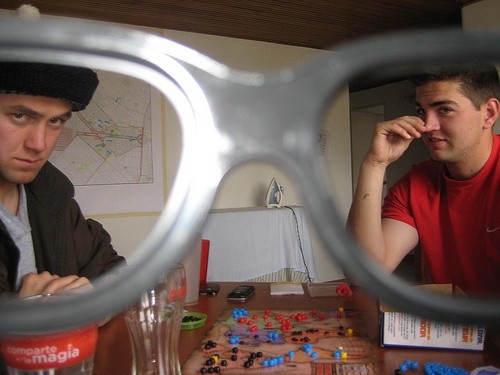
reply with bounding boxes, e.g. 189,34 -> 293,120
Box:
95,281 -> 487,375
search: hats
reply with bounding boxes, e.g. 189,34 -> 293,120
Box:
0,4 -> 100,112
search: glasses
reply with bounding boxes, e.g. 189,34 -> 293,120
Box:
0,7 -> 499,336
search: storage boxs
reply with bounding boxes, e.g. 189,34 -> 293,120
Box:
378,284 -> 486,352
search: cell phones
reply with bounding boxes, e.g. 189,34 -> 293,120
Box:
228,285 -> 255,300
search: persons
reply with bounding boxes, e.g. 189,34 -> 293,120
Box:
0,55 -> 140,327
342,56 -> 500,302
